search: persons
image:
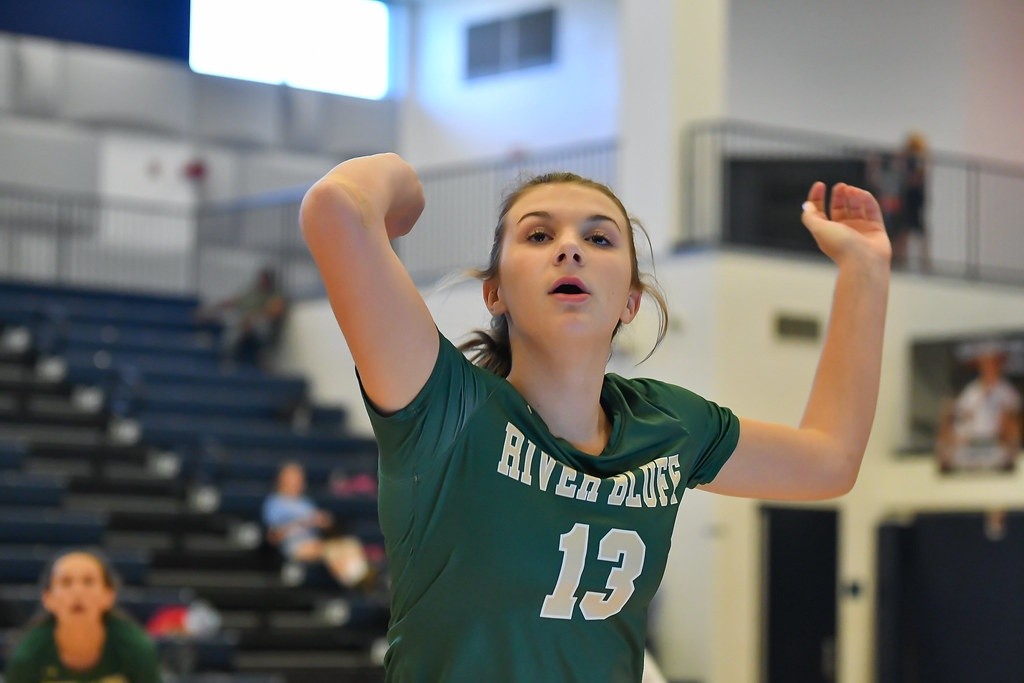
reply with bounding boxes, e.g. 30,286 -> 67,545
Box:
197,268 -> 287,370
1,548 -> 164,683
297,152 -> 890,683
263,464 -> 374,590
936,348 -> 1021,540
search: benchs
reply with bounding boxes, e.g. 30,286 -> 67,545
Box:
0,277 -> 395,683
